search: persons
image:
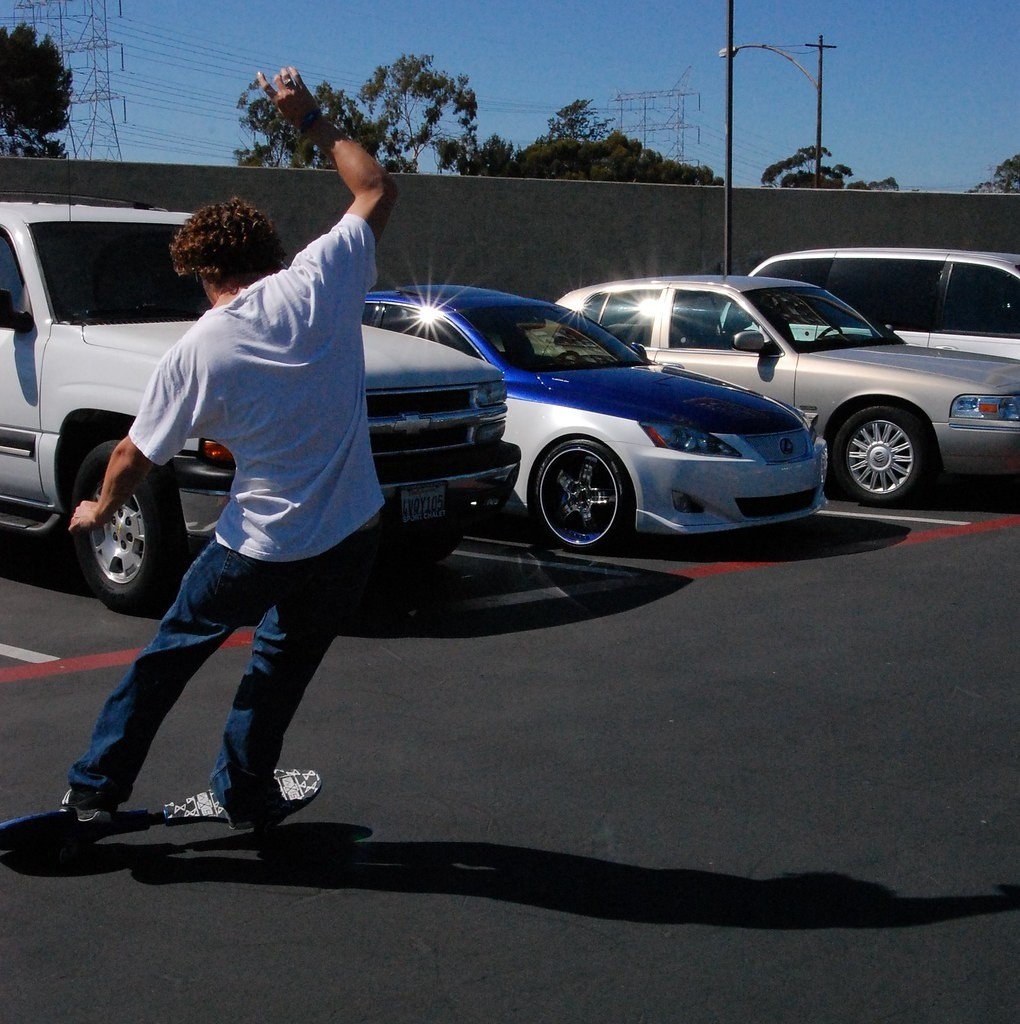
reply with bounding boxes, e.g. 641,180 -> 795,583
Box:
55,64 -> 401,833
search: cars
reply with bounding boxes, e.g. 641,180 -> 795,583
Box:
491,274 -> 1019,507
359,283 -> 830,553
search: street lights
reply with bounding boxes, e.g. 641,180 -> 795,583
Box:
719,33 -> 837,189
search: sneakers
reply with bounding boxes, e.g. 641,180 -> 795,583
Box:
61,783 -> 119,823
224,800 -> 261,833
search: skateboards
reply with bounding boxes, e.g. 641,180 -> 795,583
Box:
1,766 -> 324,854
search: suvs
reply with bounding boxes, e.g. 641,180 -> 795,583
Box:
0,189 -> 522,619
713,248 -> 1020,363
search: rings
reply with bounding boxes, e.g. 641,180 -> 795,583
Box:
282,78 -> 294,87
